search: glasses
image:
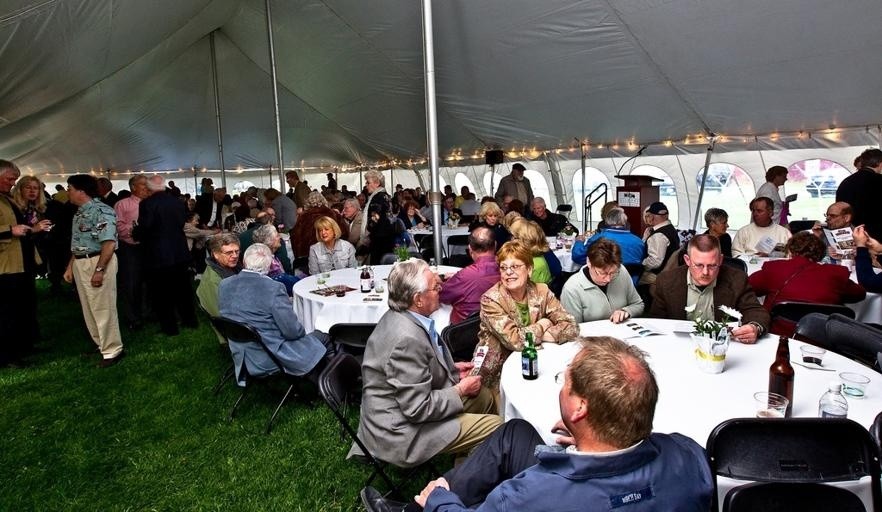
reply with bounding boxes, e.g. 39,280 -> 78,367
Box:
690,261 -> 720,272
594,267 -> 620,278
824,214 -> 841,218
499,264 -> 525,272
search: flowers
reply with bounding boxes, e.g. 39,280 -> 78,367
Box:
559,229 -> 576,240
684,304 -> 744,340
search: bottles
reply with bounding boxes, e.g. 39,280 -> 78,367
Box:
556,233 -> 563,249
521,332 -> 538,380
749,255 -> 758,264
841,251 -> 852,273
818,383 -> 848,418
428,258 -> 437,273
360,265 -> 372,293
367,266 -> 375,289
768,335 -> 795,418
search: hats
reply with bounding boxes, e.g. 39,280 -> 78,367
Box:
646,201 -> 668,215
513,163 -> 526,171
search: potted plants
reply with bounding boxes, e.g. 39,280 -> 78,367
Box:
393,244 -> 409,265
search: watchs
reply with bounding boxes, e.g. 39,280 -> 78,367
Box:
750,322 -> 760,338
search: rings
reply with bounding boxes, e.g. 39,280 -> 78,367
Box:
747,339 -> 749,343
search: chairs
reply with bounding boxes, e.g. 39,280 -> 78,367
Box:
624,262 -> 645,289
788,220 -> 815,233
448,233 -> 473,269
441,315 -> 480,362
554,204 -> 572,221
768,301 -> 882,376
413,234 -> 448,264
355,244 -> 372,265
209,316 -> 331,429
707,419 -> 882,512
867,413 -> 882,446
329,323 -> 377,437
316,350 -> 441,504
293,255 -> 309,279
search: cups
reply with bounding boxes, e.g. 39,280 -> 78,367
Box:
428,227 -> 433,232
334,283 -> 346,297
319,263 -> 332,278
800,344 -> 827,366
549,243 -> 556,250
317,272 -> 326,284
753,392 -> 790,418
564,240 -> 572,252
375,280 -> 385,293
839,372 -> 871,399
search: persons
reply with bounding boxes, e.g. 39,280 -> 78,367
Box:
360,260 -> 505,469
361,336 -> 715,512
1,159 -> 199,368
560,238 -> 645,323
572,201 -> 681,285
193,169 -> 395,273
396,162 -> 569,324
217,243 -> 335,386
702,149 -> 882,314
197,232 -> 240,317
252,224 -> 305,294
649,235 -> 771,344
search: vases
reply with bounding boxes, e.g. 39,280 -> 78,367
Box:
689,333 -> 729,374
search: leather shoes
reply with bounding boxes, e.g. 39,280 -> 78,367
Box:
362,486 -> 408,512
84,347 -> 99,357
100,350 -> 124,367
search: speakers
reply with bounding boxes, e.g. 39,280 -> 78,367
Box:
486,150 -> 503,164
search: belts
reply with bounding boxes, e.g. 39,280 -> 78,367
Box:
74,251 -> 102,259
